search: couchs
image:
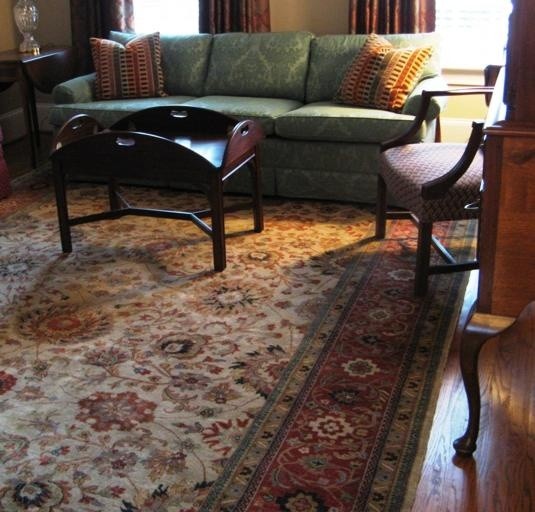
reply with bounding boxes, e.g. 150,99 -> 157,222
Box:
49,30 -> 449,208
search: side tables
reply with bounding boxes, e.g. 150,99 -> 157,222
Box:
0,47 -> 76,167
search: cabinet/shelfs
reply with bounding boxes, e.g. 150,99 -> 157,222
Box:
450,116 -> 534,456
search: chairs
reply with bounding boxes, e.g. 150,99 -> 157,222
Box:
374,65 -> 508,298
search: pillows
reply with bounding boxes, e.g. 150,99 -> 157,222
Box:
89,31 -> 169,100
333,30 -> 437,114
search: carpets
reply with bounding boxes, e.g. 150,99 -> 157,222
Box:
1,181 -> 476,511
